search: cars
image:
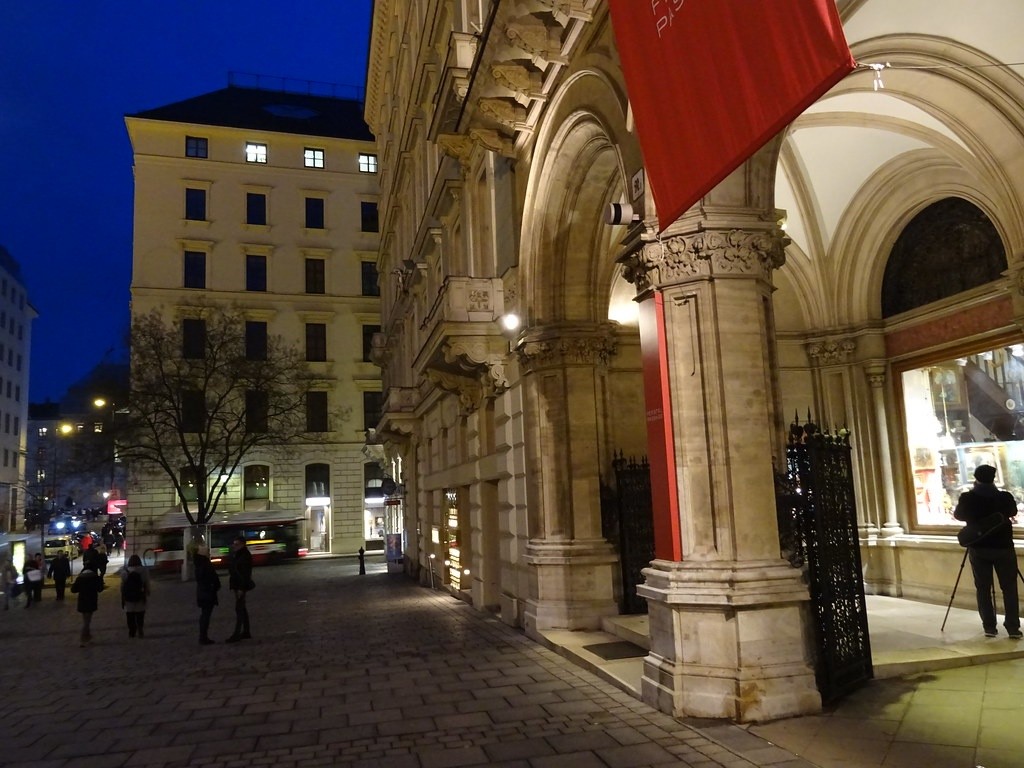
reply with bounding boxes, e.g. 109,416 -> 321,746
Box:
43,534 -> 78,561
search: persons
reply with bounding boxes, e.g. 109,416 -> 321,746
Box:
193,544 -> 222,644
225,536 -> 257,644
951,463 -> 1023,639
0,505 -> 126,647
120,555 -> 151,640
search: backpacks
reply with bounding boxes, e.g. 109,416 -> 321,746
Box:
123,567 -> 145,602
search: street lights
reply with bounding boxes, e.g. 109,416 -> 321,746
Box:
35,425 -> 49,525
52,422 -> 74,514
93,397 -> 116,489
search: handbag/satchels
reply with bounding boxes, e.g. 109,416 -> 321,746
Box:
241,580 -> 256,591
956,511 -> 1004,546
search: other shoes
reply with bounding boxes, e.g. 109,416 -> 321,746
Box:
984,628 -> 999,636
1008,630 -> 1023,640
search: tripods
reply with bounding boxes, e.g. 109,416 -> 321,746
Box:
942,548 -> 1024,631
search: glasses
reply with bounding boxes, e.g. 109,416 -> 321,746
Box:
232,543 -> 240,546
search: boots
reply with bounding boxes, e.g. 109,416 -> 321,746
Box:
225,615 -> 251,644
199,616 -> 215,645
79,634 -> 91,647
126,611 -> 145,639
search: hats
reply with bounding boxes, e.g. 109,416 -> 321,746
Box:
975,465 -> 997,484
128,554 -> 143,567
